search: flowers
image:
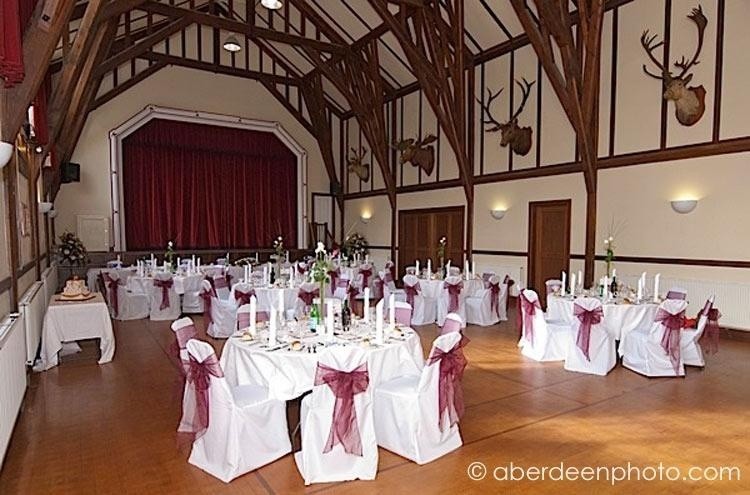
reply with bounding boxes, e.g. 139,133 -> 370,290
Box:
165,239 -> 175,261
309,240 -> 339,283
50,228 -> 92,270
601,214 -> 628,262
437,235 -> 449,258
272,236 -> 286,258
343,233 -> 370,257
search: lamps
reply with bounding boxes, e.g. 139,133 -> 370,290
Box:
261,0 -> 283,10
223,30 -> 241,53
359,215 -> 373,226
40,200 -> 58,218
0,140 -> 16,171
670,198 -> 697,214
490,209 -> 507,220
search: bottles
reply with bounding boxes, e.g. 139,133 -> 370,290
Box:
310,304 -> 320,332
611,277 -> 618,297
342,299 -> 351,329
599,279 -> 605,296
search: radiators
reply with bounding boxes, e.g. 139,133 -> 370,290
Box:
20,282 -> 47,366
41,259 -> 60,300
0,309 -> 29,475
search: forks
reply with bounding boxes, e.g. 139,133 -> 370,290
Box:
233,313 -> 404,354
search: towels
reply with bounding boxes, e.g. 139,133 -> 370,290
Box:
414,260 -> 475,281
133,253 -> 201,276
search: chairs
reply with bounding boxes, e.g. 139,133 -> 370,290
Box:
516,268 -> 724,380
98,259 -> 514,488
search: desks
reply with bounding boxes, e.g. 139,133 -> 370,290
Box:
32,293 -> 116,377
